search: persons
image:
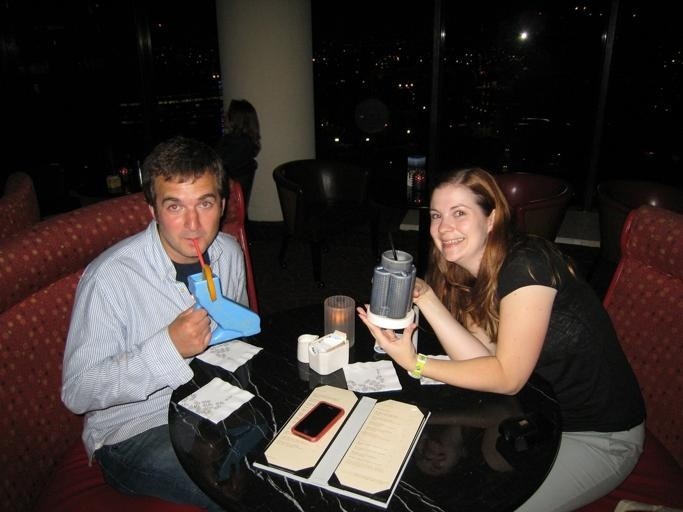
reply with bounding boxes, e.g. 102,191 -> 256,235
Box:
356,166 -> 647,511
220,99 -> 262,159
59,138 -> 250,512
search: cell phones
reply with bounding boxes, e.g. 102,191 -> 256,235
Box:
292,401 -> 345,442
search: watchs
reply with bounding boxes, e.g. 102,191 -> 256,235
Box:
407,353 -> 428,379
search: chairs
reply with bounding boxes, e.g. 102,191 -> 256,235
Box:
0,157 -> 261,510
504,171 -> 682,510
273,157 -> 405,289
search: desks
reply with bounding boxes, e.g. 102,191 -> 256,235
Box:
401,201 -> 602,248
171,300 -> 562,512
